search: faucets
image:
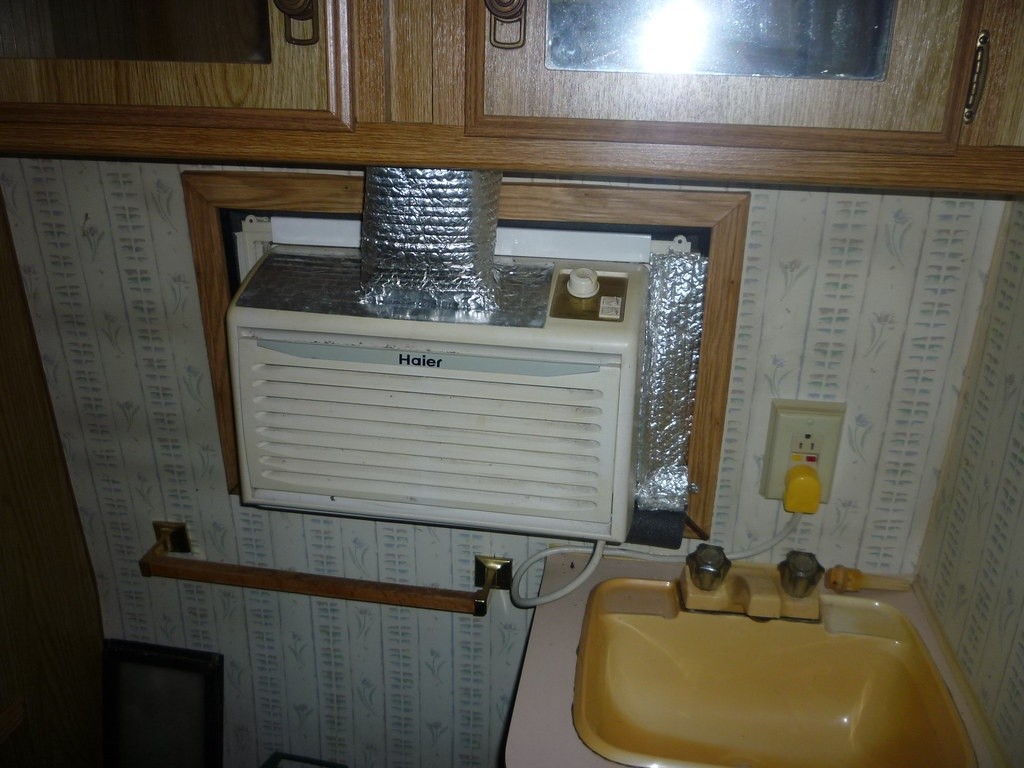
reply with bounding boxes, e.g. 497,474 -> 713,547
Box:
728,565 -> 783,624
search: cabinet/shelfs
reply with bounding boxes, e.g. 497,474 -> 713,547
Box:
0,0 -> 1024,197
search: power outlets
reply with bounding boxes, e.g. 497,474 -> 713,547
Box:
758,399 -> 848,504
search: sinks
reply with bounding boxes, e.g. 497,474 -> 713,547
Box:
569,577 -> 979,767
